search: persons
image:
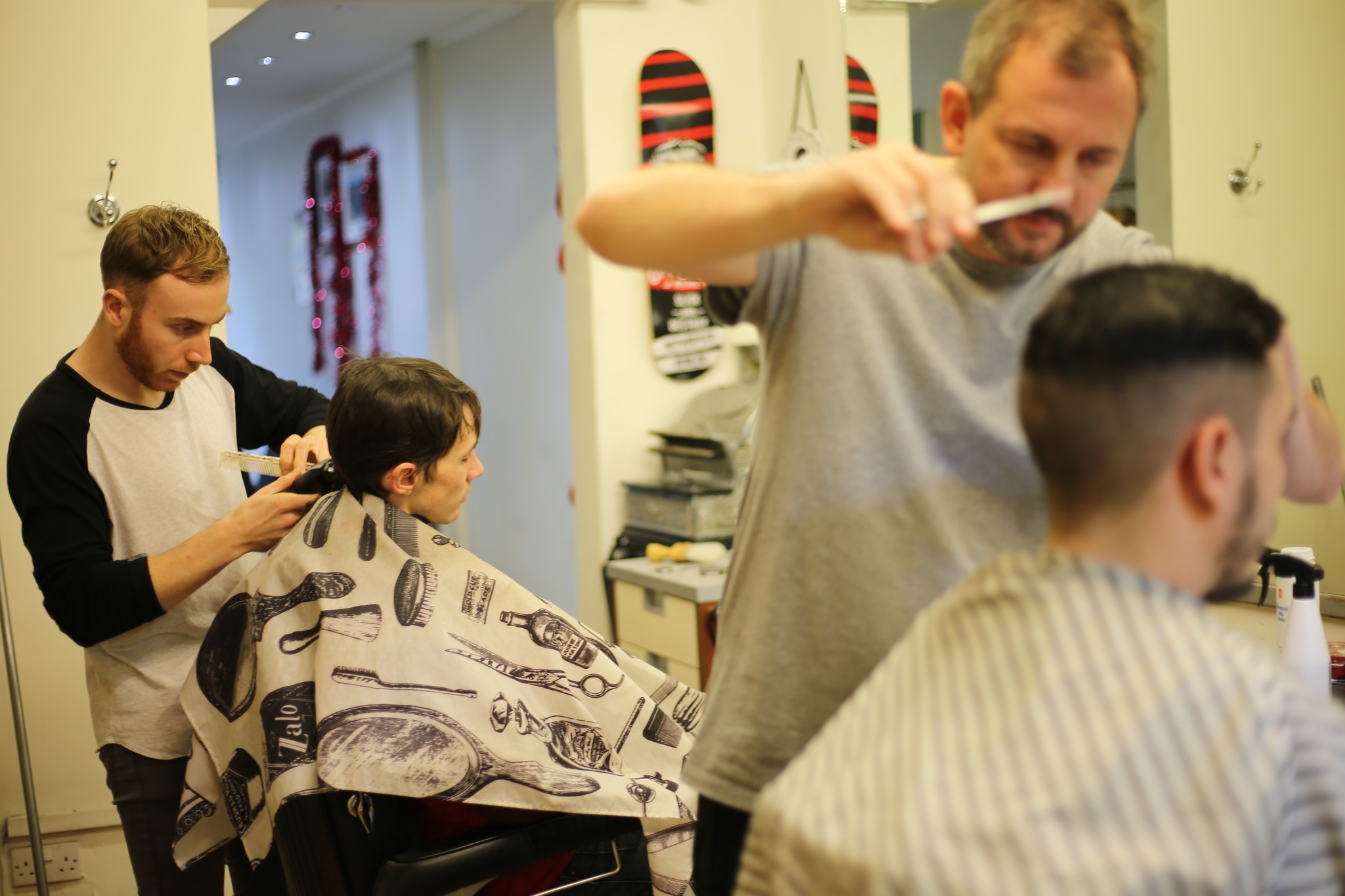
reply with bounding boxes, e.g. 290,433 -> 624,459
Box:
578,1 -> 1344,894
735,256 -> 1343,895
246,354 -> 659,896
4,207 -> 334,896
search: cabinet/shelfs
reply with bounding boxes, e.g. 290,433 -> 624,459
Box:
607,554 -> 734,692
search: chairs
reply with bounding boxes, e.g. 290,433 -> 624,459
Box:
273,782 -> 656,896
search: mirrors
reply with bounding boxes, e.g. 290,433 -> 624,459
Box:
906,0 -> 1138,227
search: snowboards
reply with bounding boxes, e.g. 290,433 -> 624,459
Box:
635,50 -> 725,382
845,49 -> 882,156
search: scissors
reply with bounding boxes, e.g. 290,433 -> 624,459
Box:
908,188 -> 1072,240
